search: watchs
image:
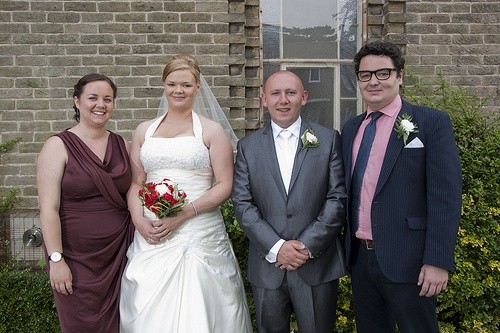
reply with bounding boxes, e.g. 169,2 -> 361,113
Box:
48,252 -> 64,262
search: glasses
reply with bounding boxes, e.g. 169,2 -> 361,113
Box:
355,68 -> 397,82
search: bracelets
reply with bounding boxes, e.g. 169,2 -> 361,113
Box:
191,202 -> 198,216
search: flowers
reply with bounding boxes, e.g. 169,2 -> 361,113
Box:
137,178 -> 188,219
393,113 -> 418,147
301,128 -> 320,150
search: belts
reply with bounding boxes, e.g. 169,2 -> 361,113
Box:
353,236 -> 375,252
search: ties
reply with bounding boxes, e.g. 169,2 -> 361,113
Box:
278,130 -> 293,194
350,112 -> 383,238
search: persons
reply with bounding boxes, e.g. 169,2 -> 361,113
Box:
341,42 -> 462,333
37,74 -> 135,333
119,54 -> 253,333
232,70 -> 347,333
